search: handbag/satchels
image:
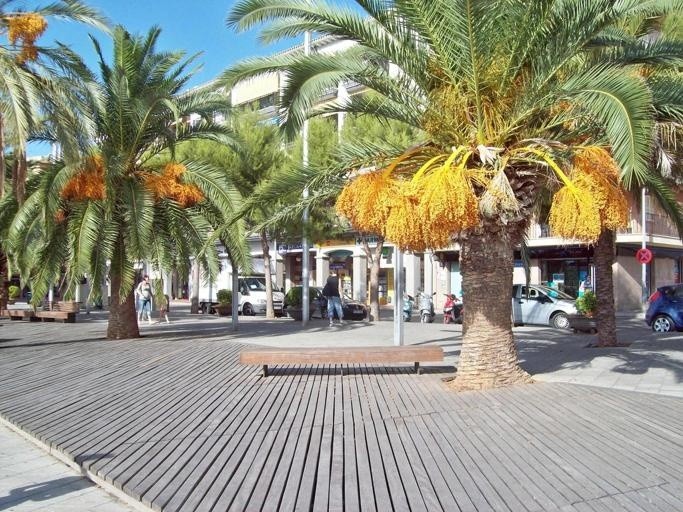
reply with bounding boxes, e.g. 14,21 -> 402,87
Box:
322,284 -> 330,296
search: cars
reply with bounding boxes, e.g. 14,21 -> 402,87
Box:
644,282 -> 683,334
282,285 -> 367,321
508,283 -> 581,330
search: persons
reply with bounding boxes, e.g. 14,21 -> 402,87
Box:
324,269 -> 349,327
159,294 -> 171,325
134,274 -> 156,325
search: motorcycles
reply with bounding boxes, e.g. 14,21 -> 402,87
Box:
403,293 -> 419,322
417,286 -> 437,324
442,293 -> 463,325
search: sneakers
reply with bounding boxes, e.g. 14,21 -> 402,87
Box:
329,321 -> 345,327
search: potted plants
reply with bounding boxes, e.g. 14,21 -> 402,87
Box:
210,288 -> 232,316
565,287 -> 598,335
283,286 -> 318,321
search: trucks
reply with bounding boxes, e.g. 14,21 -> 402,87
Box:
186,257 -> 286,316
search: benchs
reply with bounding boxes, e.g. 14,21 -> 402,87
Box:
0,301 -> 79,323
238,346 -> 444,377
